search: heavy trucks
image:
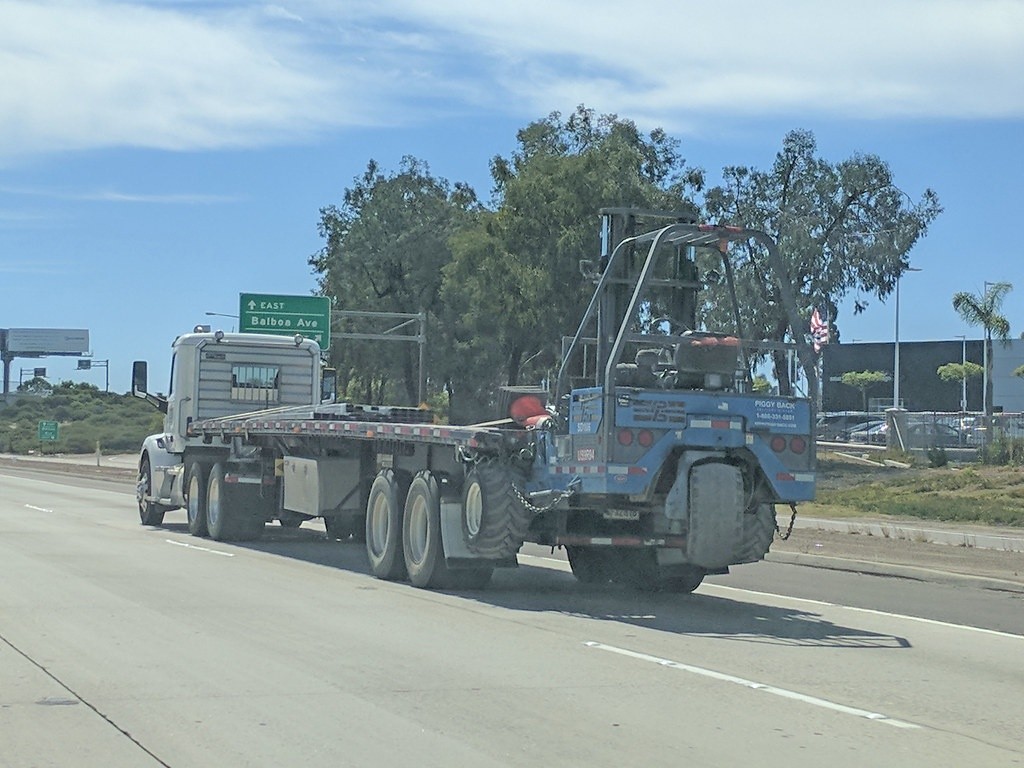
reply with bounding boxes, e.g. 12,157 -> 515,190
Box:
134,331 -> 733,598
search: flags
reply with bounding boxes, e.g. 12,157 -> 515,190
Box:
810,303 -> 829,354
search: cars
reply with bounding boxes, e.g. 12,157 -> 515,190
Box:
816,410 -> 1024,447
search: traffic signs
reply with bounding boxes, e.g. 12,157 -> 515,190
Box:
239,292 -> 331,351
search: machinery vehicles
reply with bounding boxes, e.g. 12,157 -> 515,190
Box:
460,204 -> 819,569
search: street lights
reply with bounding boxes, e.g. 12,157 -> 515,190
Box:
894,267 -> 923,408
955,335 -> 966,413
983,281 -> 996,415
